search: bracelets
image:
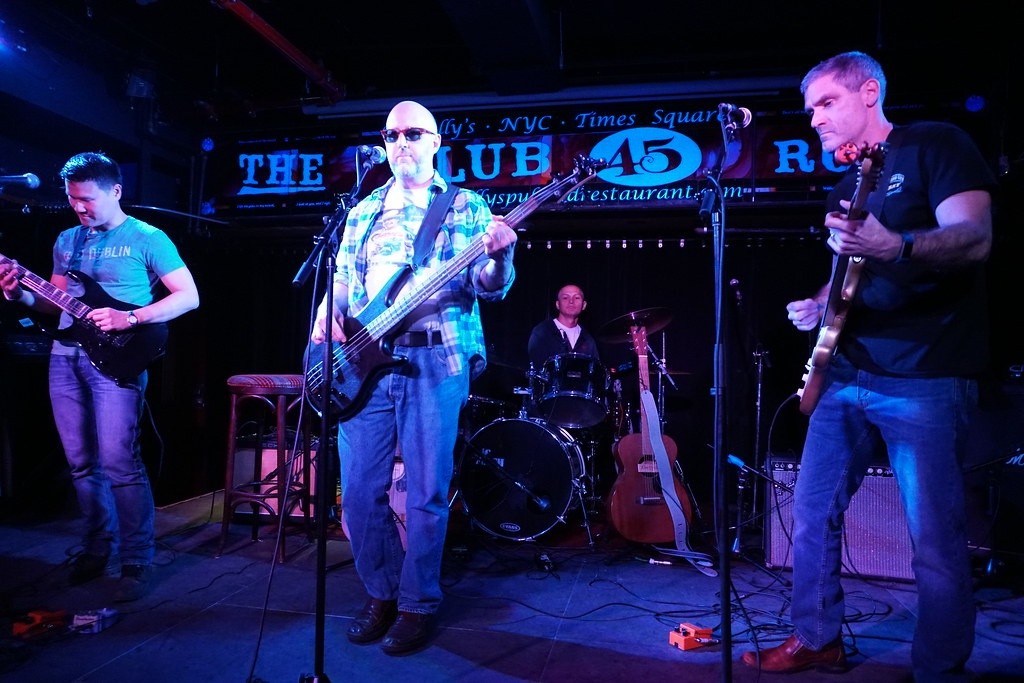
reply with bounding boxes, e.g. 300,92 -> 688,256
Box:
896,232 -> 913,263
3,291 -> 15,301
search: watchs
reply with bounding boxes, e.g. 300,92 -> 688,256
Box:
127,311 -> 138,328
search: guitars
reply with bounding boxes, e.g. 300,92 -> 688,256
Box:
0,252 -> 170,384
302,152 -> 610,420
606,326 -> 693,543
796,140 -> 892,417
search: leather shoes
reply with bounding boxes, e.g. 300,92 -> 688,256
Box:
346,597 -> 398,643
380,611 -> 439,657
743,633 -> 847,675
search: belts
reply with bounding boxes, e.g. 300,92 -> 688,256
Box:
390,331 -> 442,349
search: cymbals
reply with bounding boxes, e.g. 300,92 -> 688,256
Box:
486,352 -> 524,371
649,368 -> 695,377
595,307 -> 674,345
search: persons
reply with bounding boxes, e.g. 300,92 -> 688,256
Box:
529,285 -> 615,492
311,100 -> 517,653
0,152 -> 199,602
739,50 -> 1004,683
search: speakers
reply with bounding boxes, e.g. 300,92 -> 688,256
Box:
231,436 -> 319,527
762,456 -> 918,583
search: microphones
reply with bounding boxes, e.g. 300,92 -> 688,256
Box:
358,145 -> 386,164
0,173 -> 41,189
758,343 -> 773,368
718,103 -> 752,128
533,497 -> 551,512
609,367 -> 622,401
833,142 -> 859,165
563,331 -> 572,353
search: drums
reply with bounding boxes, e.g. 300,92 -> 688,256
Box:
524,352 -> 610,429
458,395 -> 521,447
341,459 -> 407,551
456,417 -> 586,542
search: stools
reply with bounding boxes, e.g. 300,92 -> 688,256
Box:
212,373 -> 316,564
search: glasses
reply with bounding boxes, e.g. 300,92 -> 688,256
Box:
379,126 -> 435,142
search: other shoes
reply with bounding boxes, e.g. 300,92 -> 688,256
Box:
67,549 -> 122,585
111,564 -> 153,602
